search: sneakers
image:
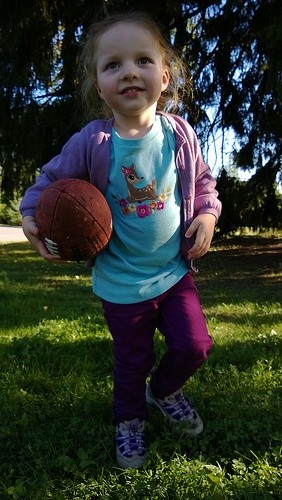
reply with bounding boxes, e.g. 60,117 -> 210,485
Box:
115,419 -> 149,469
146,383 -> 203,435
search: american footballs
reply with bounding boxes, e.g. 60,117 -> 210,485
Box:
34,176 -> 114,262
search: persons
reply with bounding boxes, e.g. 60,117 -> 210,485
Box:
18,11 -> 221,471
